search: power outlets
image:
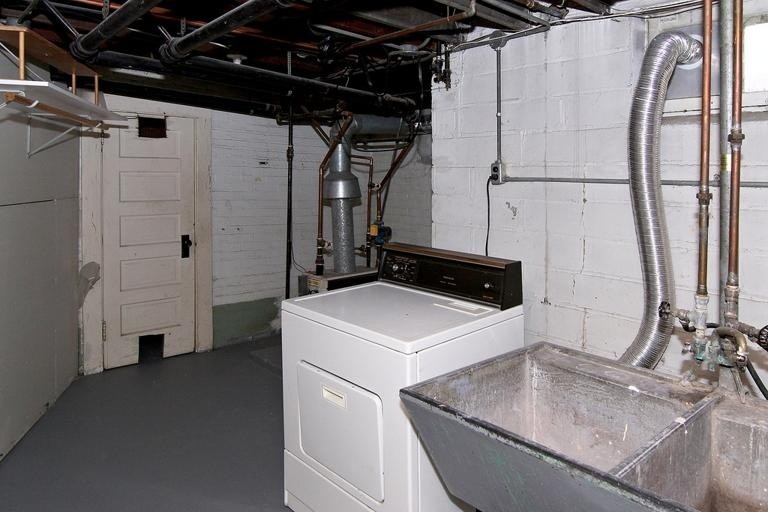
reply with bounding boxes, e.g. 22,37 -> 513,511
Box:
490,162 -> 506,186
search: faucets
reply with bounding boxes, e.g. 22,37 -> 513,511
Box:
709,324 -> 750,374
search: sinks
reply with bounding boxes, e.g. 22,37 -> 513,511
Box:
415,358 -> 692,471
620,408 -> 768,512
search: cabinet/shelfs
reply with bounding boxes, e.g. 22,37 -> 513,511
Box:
0,24 -> 127,155
